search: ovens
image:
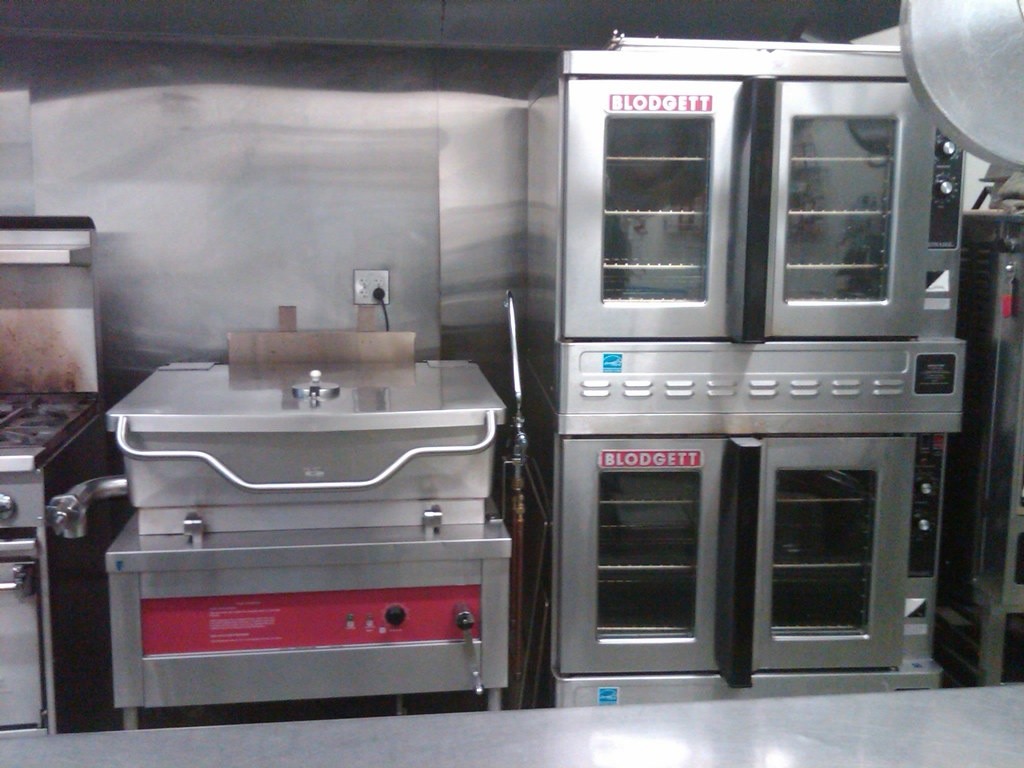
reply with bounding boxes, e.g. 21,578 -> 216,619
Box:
0,532 -> 60,742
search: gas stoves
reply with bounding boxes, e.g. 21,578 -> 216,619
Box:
0,393 -> 104,512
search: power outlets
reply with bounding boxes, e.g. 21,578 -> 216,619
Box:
353,269 -> 389,305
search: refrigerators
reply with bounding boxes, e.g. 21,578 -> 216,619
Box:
528,37 -> 965,711
